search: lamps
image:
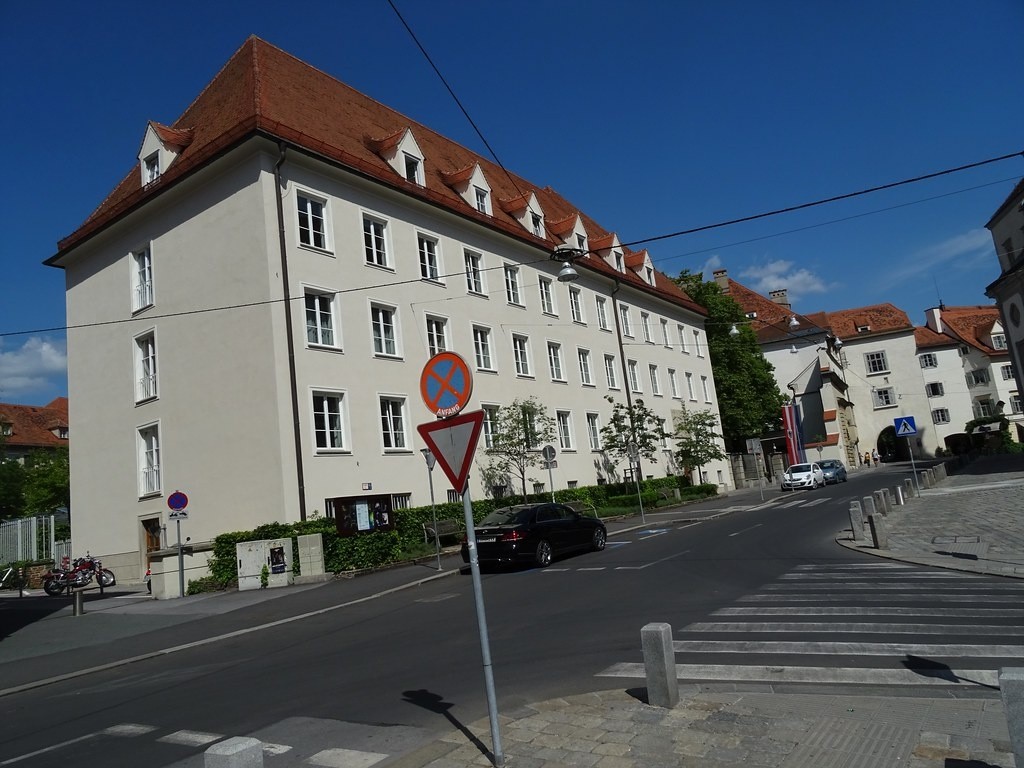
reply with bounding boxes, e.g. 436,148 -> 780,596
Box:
834,337 -> 844,349
789,316 -> 800,330
790,344 -> 799,353
729,325 -> 740,335
557,261 -> 580,282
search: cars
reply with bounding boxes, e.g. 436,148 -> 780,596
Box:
461,503 -> 607,567
781,463 -> 827,491
815,460 -> 847,485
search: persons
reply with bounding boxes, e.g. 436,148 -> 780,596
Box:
872,449 -> 878,467
864,452 -> 870,468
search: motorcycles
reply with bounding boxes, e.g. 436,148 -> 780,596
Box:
40,552 -> 115,596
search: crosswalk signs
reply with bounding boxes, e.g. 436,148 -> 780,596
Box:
893,416 -> 916,437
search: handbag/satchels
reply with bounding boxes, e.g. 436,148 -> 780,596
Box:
864,460 -> 867,464
